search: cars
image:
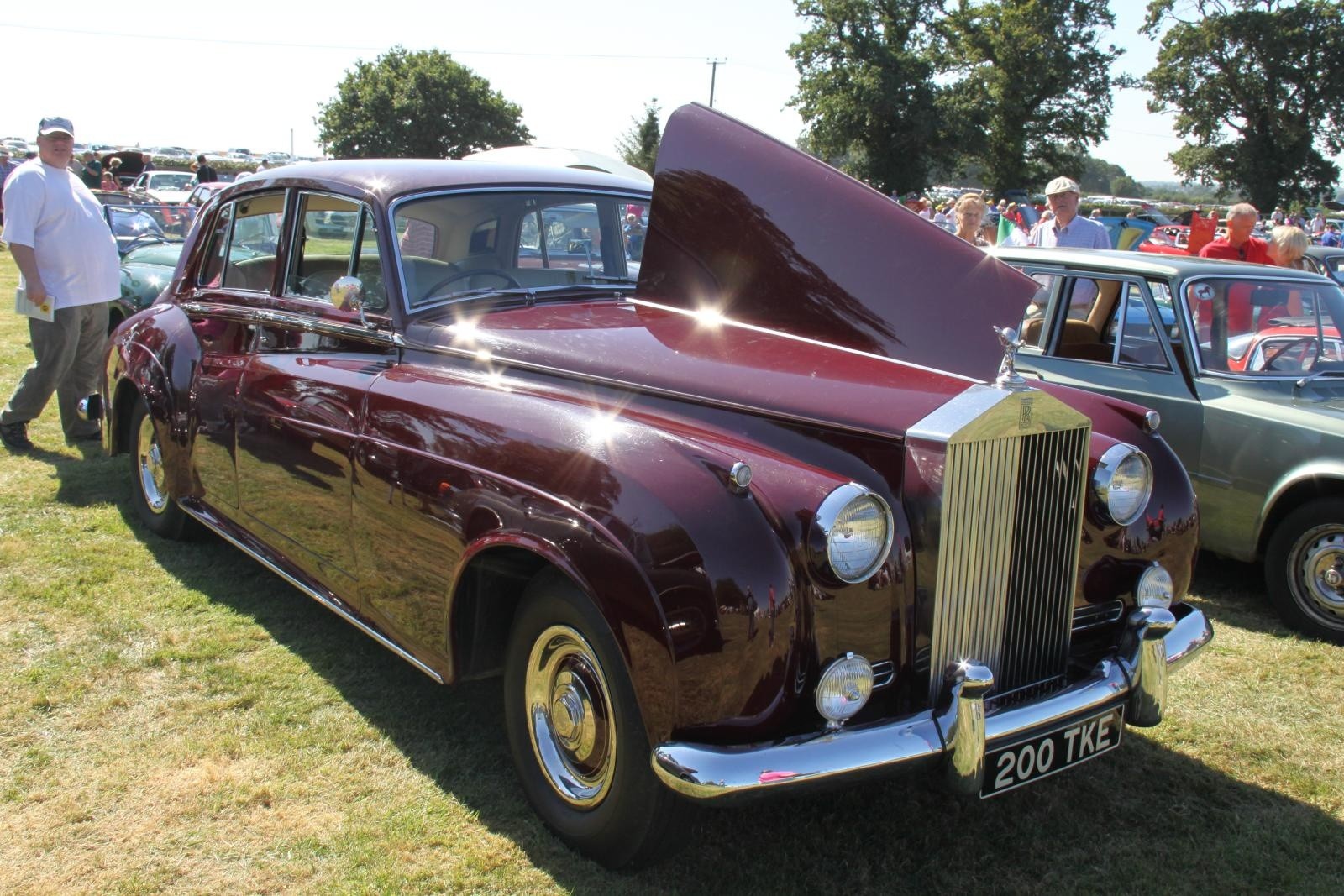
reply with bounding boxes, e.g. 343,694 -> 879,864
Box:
924,180 -> 1206,205
77,103 -> 1216,872
1089,204 -> 1344,242
86,190 -> 278,339
183,182 -> 234,207
0,135 -> 313,168
127,169 -> 198,205
305,210 -> 375,238
400,142 -> 658,279
964,241 -> 1344,649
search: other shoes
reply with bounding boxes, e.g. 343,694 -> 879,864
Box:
82,428 -> 103,440
0,420 -> 33,450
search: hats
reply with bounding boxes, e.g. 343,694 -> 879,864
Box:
1091,208 -> 1100,217
986,200 -> 994,205
935,205 -> 943,210
1045,176 -> 1081,196
0,145 -> 14,157
109,157 -> 123,166
80,151 -> 93,155
38,116 -> 74,138
1275,206 -> 1280,211
1009,202 -> 1016,208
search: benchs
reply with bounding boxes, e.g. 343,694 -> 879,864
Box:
235,253 -> 463,310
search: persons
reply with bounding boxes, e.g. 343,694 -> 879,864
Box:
868,562 -> 892,591
400,218 -> 434,258
1127,208 -> 1136,218
0,144 -> 20,227
721,605 -> 766,620
257,159 -> 267,173
0,117 -> 123,449
1028,176 -> 1112,320
890,190 -> 1052,247
768,585 -> 775,647
233,171 -> 279,255
185,154 -> 217,189
746,586 -> 755,640
141,154 -> 155,171
1306,213 -> 1344,278
1145,504 -> 1165,541
1187,203 -> 1309,343
68,150 -> 123,190
621,203 -> 646,262
1118,531 -> 1125,551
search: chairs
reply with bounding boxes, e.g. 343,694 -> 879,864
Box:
475,266 -> 578,291
1021,317 -> 1101,346
292,268 -> 386,308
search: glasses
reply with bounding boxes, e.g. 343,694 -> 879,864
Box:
40,134 -> 71,141
1240,248 -> 1246,263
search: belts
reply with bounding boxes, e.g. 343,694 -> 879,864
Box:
1034,302 -> 1090,311
